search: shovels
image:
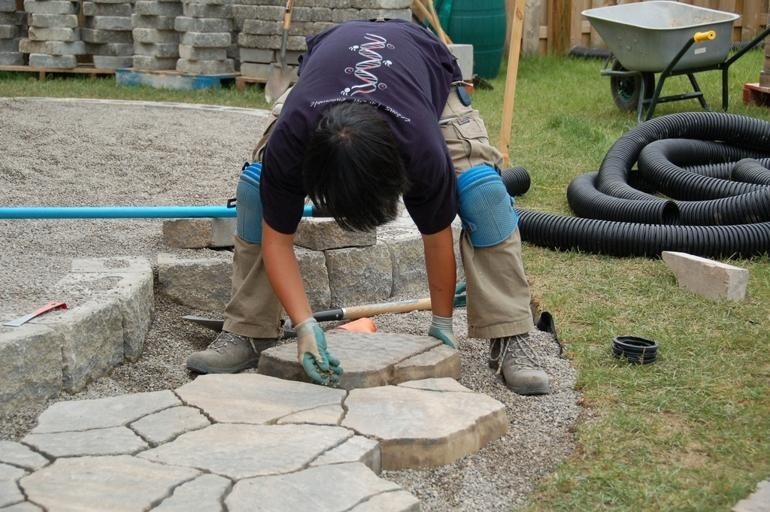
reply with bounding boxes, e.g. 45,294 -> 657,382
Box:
180,284 -> 467,340
414,0 -> 493,90
264,0 -> 295,105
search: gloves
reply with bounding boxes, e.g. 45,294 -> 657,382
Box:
294,317 -> 343,386
428,315 -> 456,349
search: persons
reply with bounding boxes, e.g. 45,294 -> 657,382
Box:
183,16 -> 551,397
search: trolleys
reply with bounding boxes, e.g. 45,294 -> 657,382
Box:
576,1 -> 768,124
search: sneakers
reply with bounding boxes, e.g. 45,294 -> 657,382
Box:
187,331 -> 276,373
489,334 -> 549,395
